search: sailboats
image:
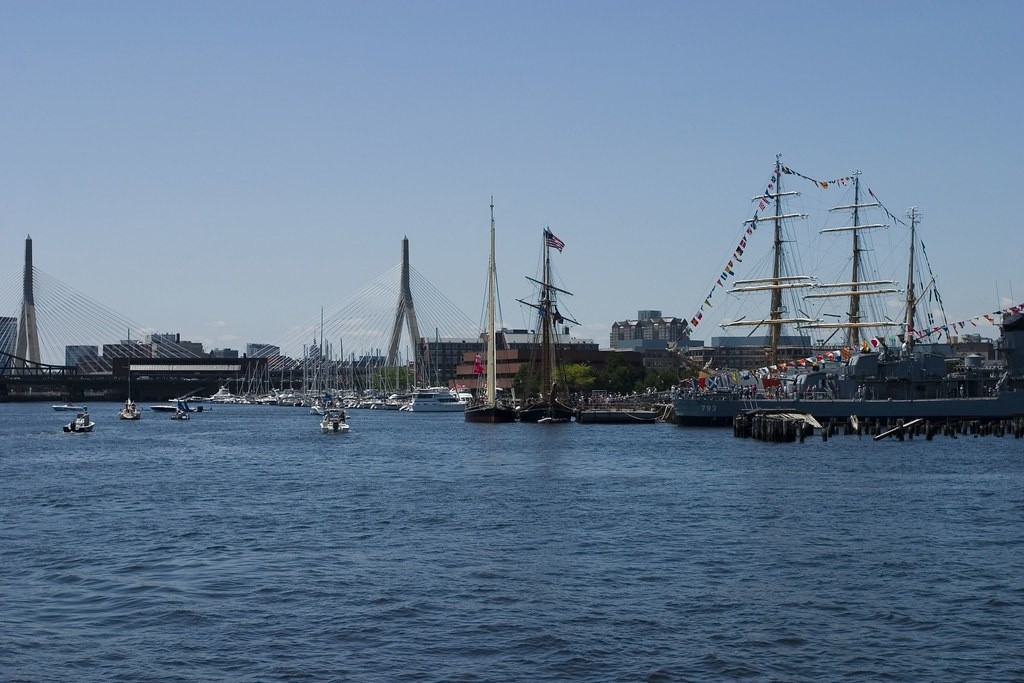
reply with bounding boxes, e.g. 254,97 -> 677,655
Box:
117,327 -> 143,421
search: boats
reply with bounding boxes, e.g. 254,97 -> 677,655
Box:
320,407 -> 351,434
148,151 -> 1024,443
63,405 -> 96,434
52,402 -> 84,413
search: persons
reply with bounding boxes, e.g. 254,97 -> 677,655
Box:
570,381 -> 996,409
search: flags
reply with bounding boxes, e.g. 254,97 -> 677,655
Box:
544,228 -> 565,253
690,303 -> 1024,391
